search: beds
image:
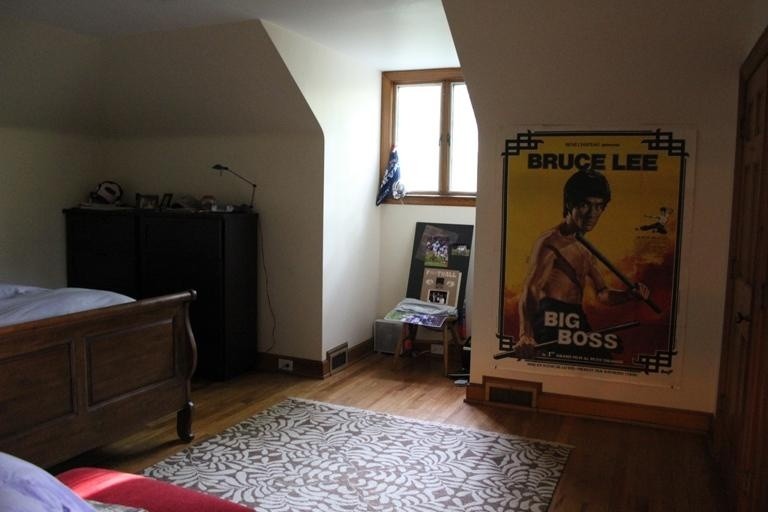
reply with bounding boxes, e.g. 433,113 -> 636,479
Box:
0,284 -> 198,471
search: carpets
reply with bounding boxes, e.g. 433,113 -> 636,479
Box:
136,396 -> 574,512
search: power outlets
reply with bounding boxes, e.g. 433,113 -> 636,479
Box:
278,359 -> 293,371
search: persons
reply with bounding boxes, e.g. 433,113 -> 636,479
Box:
383,310 -> 450,328
516,168 -> 656,359
425,240 -> 447,264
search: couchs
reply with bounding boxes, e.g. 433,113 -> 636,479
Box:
0,451 -> 255,512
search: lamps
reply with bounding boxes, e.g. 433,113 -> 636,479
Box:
211,163 -> 257,211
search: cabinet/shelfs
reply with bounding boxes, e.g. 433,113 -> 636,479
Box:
61,206 -> 257,383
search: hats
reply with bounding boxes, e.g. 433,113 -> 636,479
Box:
96,181 -> 124,204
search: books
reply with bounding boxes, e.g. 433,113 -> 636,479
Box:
423,234 -> 449,268
419,266 -> 464,316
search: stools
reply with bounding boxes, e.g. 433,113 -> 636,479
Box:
401,317 -> 462,375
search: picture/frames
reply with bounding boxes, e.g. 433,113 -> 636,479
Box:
490,123 -> 698,391
